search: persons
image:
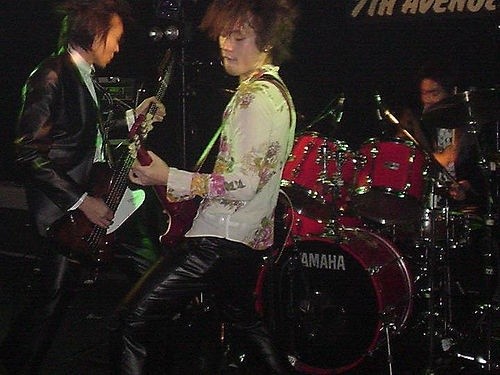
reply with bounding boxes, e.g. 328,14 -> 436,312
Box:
0,0 -> 175,375
394,57 -> 482,214
112,0 -> 303,375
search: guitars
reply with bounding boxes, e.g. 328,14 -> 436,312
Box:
49,48 -> 179,262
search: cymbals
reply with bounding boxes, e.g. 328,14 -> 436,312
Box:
422,88 -> 500,129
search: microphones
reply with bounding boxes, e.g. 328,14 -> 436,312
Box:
335,93 -> 345,122
374,94 -> 383,121
297,300 -> 339,313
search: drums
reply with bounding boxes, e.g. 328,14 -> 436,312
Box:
251,223 -> 415,370
357,137 -> 429,226
281,132 -> 363,213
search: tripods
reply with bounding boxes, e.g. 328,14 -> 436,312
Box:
380,95 -> 500,375
